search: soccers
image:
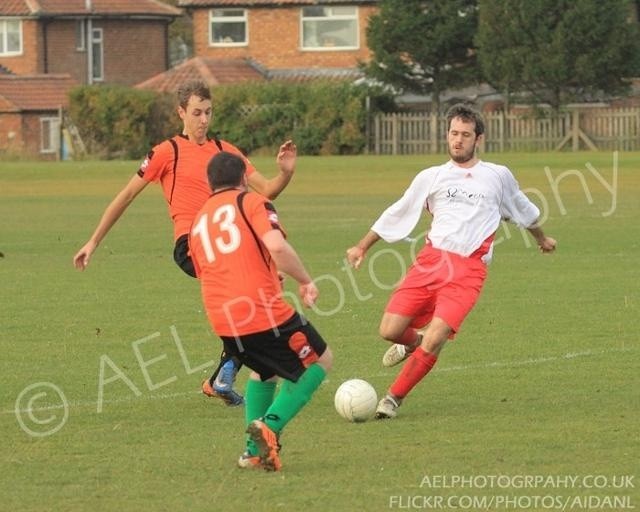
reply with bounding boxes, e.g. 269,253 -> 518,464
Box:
335,379 -> 377,422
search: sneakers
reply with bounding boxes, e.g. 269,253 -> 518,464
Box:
382,331 -> 424,367
375,391 -> 401,418
246,417 -> 282,472
238,450 -> 266,471
202,379 -> 244,407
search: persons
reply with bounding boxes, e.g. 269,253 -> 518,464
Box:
188,151 -> 333,472
344,104 -> 558,418
72,79 -> 297,405
